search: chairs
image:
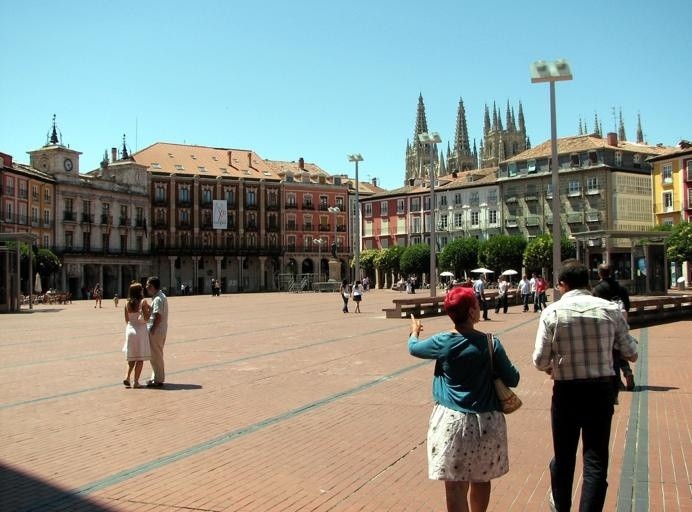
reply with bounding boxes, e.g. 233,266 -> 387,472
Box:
20,292 -> 72,304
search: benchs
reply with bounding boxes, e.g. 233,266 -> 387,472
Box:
381,287 -> 546,318
629,295 -> 692,330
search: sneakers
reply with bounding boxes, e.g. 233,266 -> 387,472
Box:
123,379 -> 163,388
626,372 -> 634,390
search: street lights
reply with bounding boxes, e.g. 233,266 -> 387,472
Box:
528,57 -> 574,304
418,131 -> 442,297
348,153 -> 364,283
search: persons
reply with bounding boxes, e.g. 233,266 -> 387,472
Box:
211,278 -> 216,296
464,272 -> 549,321
531,256 -> 642,512
340,278 -> 351,313
215,280 -> 220,296
400,273 -> 417,293
363,276 -> 368,290
123,282 -> 153,388
180,283 -> 185,296
406,288 -> 521,512
93,282 -> 103,308
352,280 -> 365,313
113,293 -> 119,307
591,263 -> 636,392
145,276 -> 168,387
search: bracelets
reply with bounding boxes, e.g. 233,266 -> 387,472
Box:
409,331 -> 420,336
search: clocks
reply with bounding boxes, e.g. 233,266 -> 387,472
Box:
64,159 -> 73,171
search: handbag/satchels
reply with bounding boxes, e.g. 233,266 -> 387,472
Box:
487,332 -> 522,414
343,292 -> 351,299
137,299 -> 150,324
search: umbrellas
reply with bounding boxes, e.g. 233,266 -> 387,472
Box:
440,272 -> 454,277
502,269 -> 518,282
470,268 -> 494,274
